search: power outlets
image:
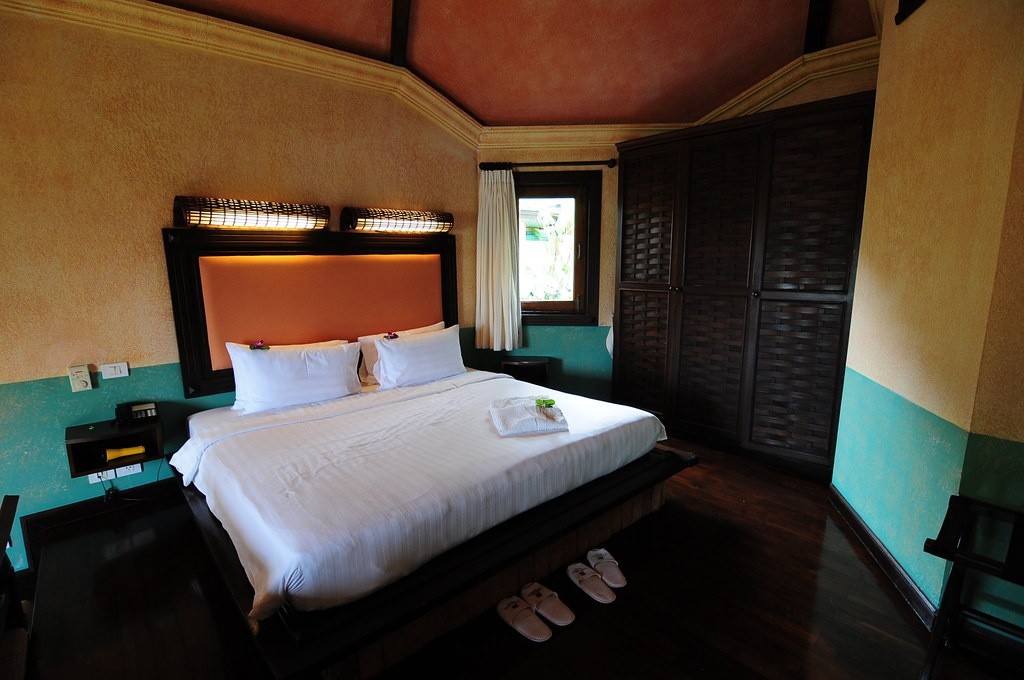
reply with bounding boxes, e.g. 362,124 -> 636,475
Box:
87,469 -> 116,485
116,464 -> 142,478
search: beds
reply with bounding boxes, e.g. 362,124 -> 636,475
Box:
163,228 -> 699,680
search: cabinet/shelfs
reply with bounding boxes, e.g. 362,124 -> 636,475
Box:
610,90 -> 877,487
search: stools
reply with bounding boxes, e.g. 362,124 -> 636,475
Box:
917,495 -> 1024,680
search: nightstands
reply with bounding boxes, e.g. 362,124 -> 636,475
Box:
65,419 -> 165,479
502,356 -> 550,385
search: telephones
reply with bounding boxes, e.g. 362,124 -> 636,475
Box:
116,401 -> 160,430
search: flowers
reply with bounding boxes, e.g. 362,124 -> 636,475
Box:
250,337 -> 270,350
383,331 -> 399,339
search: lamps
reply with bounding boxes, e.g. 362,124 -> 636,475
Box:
340,207 -> 454,234
172,196 -> 331,232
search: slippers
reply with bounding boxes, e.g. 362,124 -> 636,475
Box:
568,562 -> 618,604
497,595 -> 553,642
587,548 -> 628,589
519,582 -> 575,628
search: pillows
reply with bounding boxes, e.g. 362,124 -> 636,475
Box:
358,321 -> 444,386
373,324 -> 467,391
225,340 -> 362,417
